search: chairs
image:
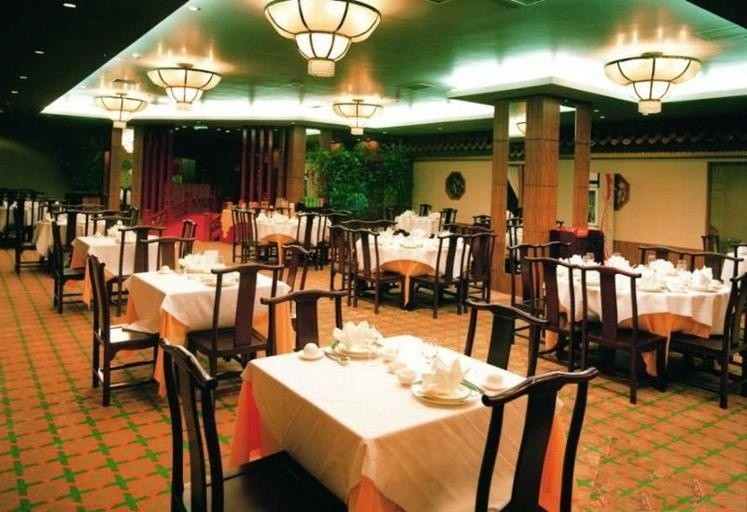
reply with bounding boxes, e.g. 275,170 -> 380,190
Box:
503,218 -> 747,409
258,284 -> 352,367
84,238 -> 318,407
327,203 -> 498,319
1,186 -> 198,312
154,332 -> 349,512
228,200 -> 352,272
473,365 -> 605,511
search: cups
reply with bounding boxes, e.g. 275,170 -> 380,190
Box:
382,340 -> 399,360
303,343 -> 318,357
388,361 -> 406,372
485,375 -> 502,385
554,253 -> 722,296
421,337 -> 439,370
393,369 -> 416,386
160,265 -> 169,272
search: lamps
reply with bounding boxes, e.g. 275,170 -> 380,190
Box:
603,50 -> 702,115
145,59 -> 225,111
93,88 -> 152,134
259,1 -> 384,80
333,93 -> 383,136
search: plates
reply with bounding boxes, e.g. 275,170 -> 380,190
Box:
412,382 -> 479,404
297,349 -> 324,360
481,381 -> 506,390
368,226 -> 449,249
179,264 -> 243,291
157,271 -> 172,274
335,342 -> 381,359
